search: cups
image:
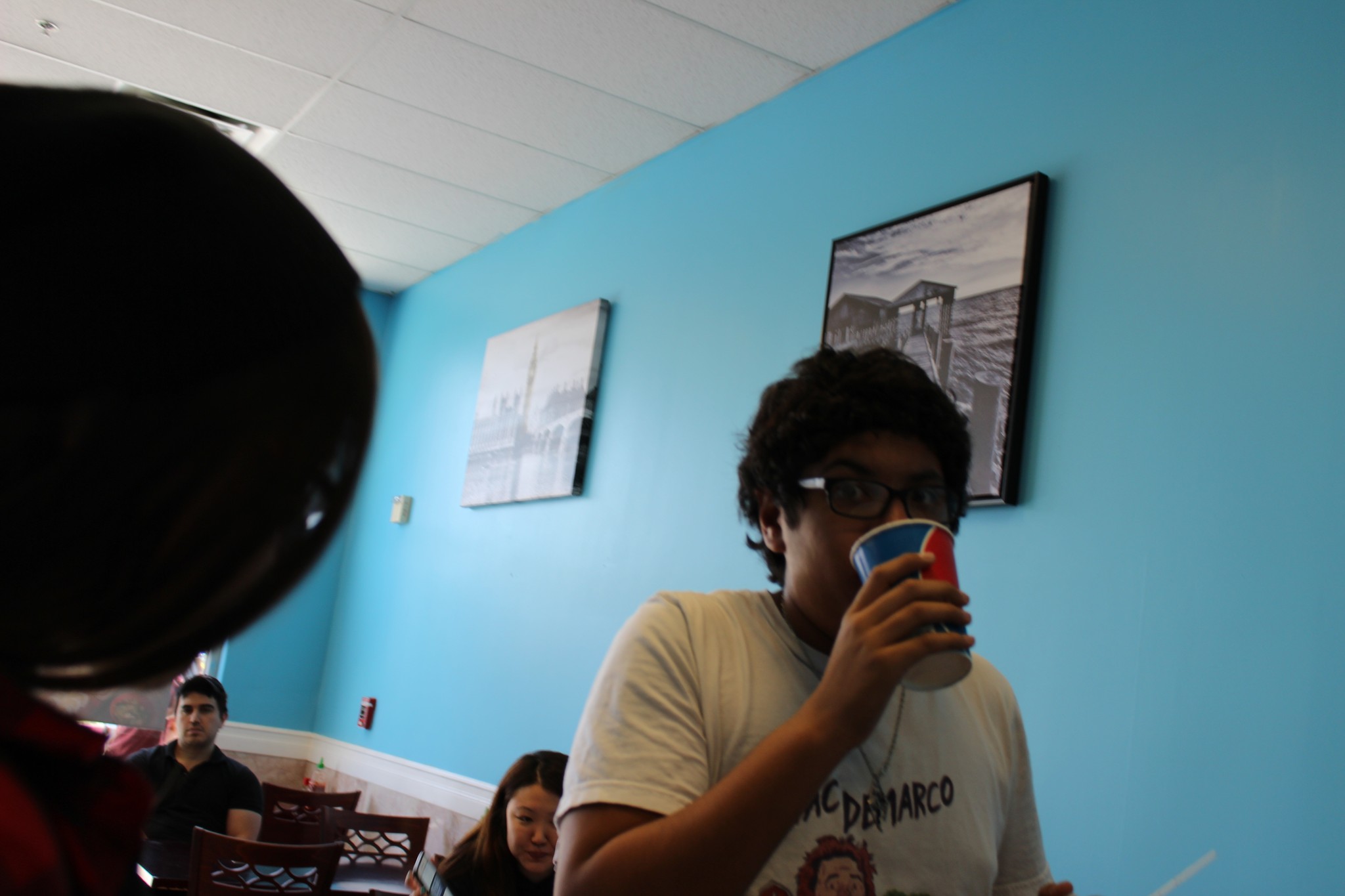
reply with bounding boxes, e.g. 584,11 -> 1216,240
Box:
850,518 -> 972,693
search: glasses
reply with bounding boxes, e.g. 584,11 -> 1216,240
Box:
797,478 -> 958,526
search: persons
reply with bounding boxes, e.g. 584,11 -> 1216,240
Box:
119,673 -> 263,894
440,748 -> 570,894
545,344 -> 1073,896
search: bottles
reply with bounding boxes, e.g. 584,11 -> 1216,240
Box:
304,756 -> 327,793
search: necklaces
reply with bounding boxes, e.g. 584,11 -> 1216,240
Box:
779,594 -> 906,806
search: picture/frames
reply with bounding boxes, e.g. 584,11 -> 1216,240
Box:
819,170 -> 1051,508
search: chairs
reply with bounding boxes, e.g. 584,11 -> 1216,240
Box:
188,825 -> 343,896
317,802 -> 428,865
262,780 -> 361,842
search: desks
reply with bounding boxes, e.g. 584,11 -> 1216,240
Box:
136,840 -> 418,896
